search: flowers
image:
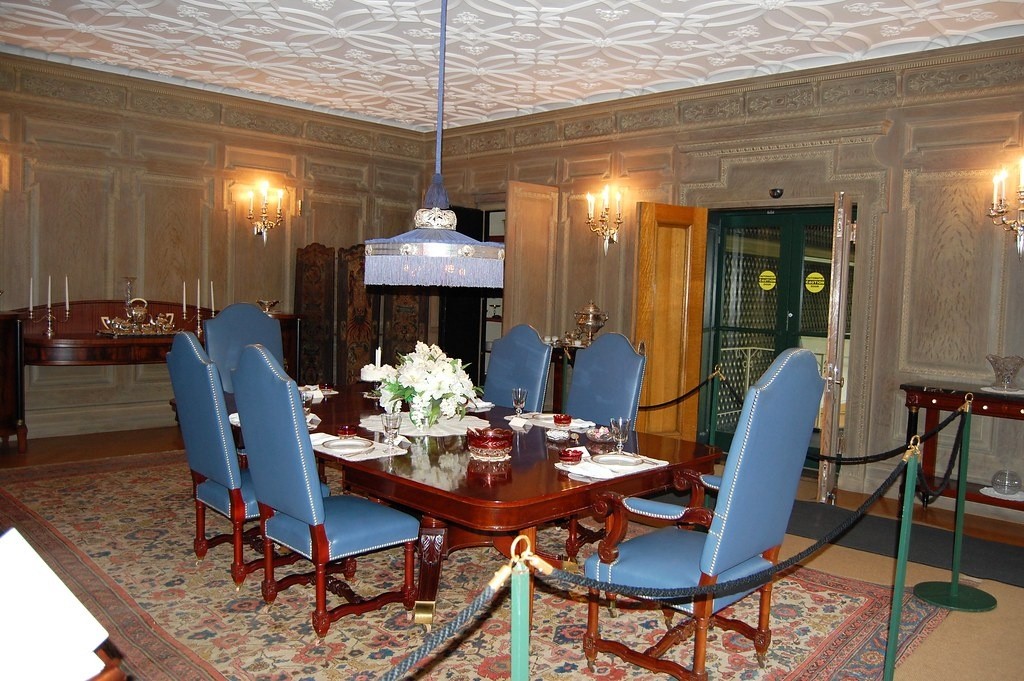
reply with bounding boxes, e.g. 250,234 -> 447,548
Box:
366,341 -> 483,426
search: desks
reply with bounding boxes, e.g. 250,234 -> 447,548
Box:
170,382 -> 724,645
899,378 -> 1024,520
549,345 -> 580,412
22,333 -> 205,366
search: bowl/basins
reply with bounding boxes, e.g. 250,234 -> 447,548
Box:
337,425 -> 358,439
554,414 -> 573,426
558,449 -> 582,466
585,428 -> 614,443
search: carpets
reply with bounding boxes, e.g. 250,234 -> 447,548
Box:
0,450 -> 953,680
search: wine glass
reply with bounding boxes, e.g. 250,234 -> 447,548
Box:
609,416 -> 631,456
512,388 -> 528,419
381,413 -> 403,454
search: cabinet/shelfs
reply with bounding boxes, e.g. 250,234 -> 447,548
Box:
485,295 -> 503,353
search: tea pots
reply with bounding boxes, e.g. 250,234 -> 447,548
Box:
110,298 -> 174,334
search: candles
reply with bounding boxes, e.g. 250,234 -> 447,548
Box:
182,280 -> 186,311
30,277 -> 33,311
47,275 -> 51,308
376,346 -> 382,367
210,280 -> 215,311
197,277 -> 201,309
65,275 -> 69,310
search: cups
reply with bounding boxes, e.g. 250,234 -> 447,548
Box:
257,298 -> 280,311
300,392 -> 314,415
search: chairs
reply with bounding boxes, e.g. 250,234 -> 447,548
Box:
565,331 -> 647,557
166,302 -> 420,638
483,323 -> 553,412
582,349 -> 827,680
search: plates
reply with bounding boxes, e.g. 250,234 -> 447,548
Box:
590,453 -> 644,468
321,390 -> 339,395
322,438 -> 373,450
533,413 -> 559,423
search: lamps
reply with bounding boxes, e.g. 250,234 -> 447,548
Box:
247,188 -> 285,245
986,163 -> 1024,260
364,0 -> 506,289
585,184 -> 623,256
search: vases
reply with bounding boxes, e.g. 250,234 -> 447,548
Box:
423,417 -> 439,425
